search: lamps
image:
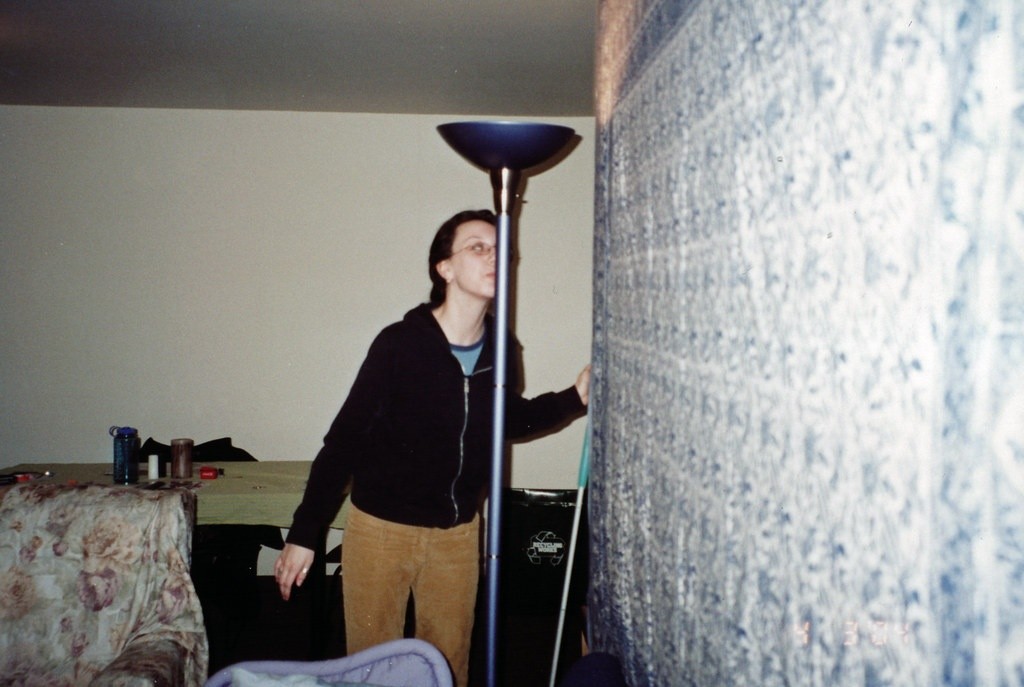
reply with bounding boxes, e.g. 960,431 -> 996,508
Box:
436,121 -> 574,687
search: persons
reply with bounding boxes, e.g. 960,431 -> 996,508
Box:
275,208 -> 591,687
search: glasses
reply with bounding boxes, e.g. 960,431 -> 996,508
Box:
453,243 -> 497,256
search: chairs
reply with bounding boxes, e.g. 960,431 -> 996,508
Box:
0,485 -> 209,687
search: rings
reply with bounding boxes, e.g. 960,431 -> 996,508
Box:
278,570 -> 282,575
302,567 -> 308,573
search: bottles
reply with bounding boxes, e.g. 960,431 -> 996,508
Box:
110,425 -> 141,483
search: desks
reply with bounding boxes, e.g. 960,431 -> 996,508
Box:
0,460 -> 348,660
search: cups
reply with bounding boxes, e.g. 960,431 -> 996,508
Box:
170,438 -> 193,479
